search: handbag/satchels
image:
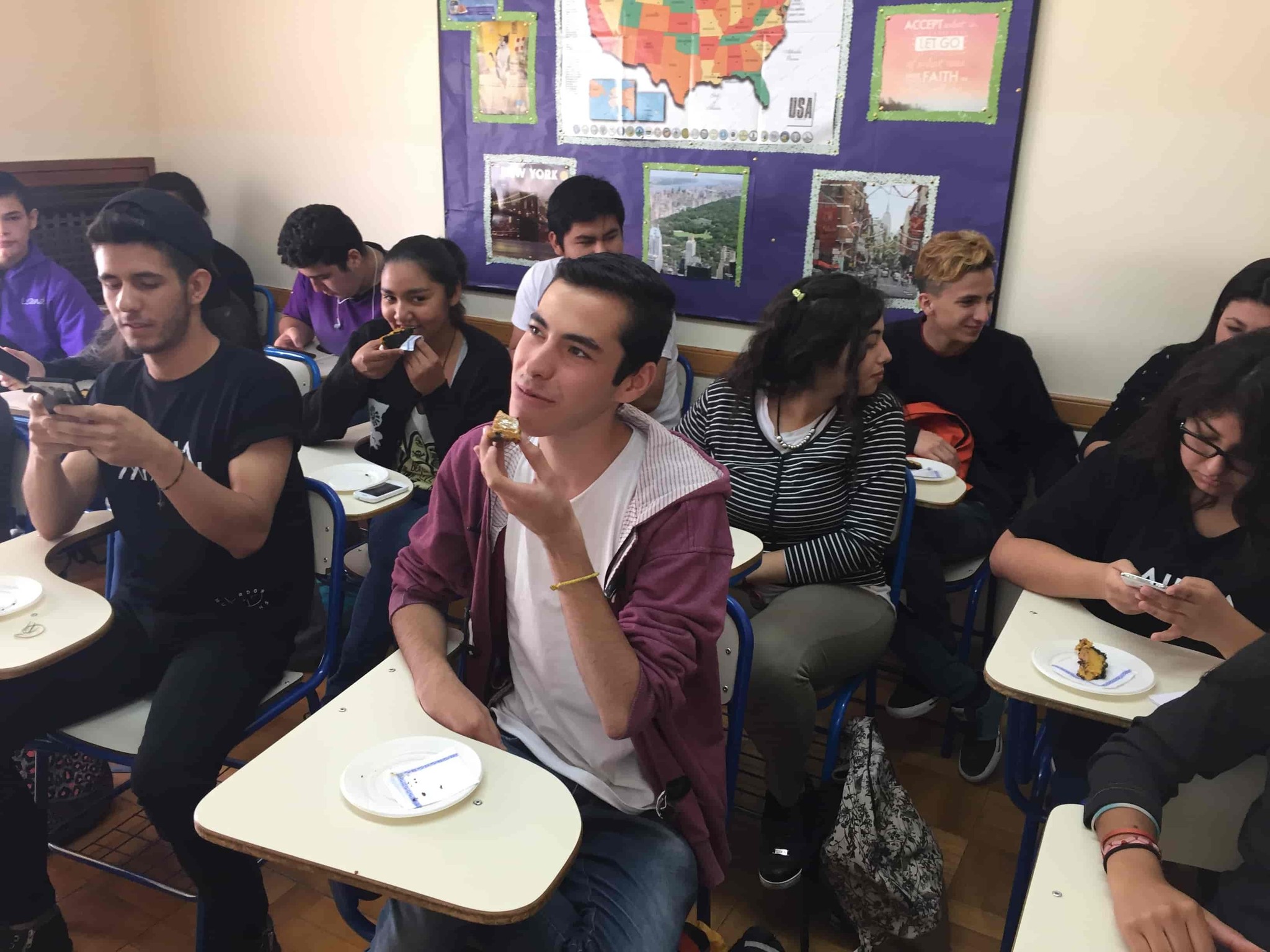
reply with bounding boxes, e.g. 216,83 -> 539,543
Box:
14,742 -> 112,845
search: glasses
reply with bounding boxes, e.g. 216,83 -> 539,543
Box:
1179,417 -> 1258,477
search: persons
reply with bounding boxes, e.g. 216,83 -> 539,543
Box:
366,252 -> 734,951
0,187 -> 315,952
510,174 -> 680,432
0,177 -> 104,363
884,229 -> 1081,783
273,204 -> 388,356
1080,258 -> 1270,461
670,274 -> 901,888
302,234 -> 511,720
0,172 -> 258,388
1083,630 -> 1270,952
989,326 -> 1270,805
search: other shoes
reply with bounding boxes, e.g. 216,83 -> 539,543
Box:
1,908 -> 72,952
196,919 -> 282,952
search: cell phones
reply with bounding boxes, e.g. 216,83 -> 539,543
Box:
0,348 -> 31,388
266,345 -> 316,359
1120,572 -> 1168,595
27,375 -> 88,415
353,478 -> 409,504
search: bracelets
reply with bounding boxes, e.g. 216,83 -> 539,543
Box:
549,572 -> 600,591
158,451 -> 186,491
1099,828 -> 1163,874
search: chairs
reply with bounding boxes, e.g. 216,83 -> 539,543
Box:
0,284 -> 1270,952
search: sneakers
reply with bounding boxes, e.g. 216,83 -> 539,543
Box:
886,675 -> 940,718
758,837 -> 802,891
958,670 -> 1010,782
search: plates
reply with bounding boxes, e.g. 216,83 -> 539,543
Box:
905,457 -> 957,480
0,574 -> 43,617
340,736 -> 485,821
1030,641 -> 1157,696
307,463 -> 390,492
